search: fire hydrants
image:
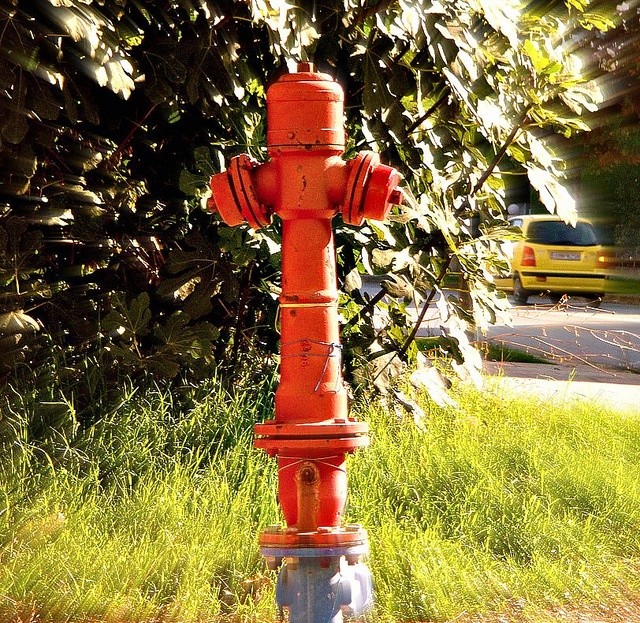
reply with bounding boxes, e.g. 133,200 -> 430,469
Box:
206,60 -> 406,623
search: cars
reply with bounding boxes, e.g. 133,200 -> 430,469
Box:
482,214 -> 610,309
